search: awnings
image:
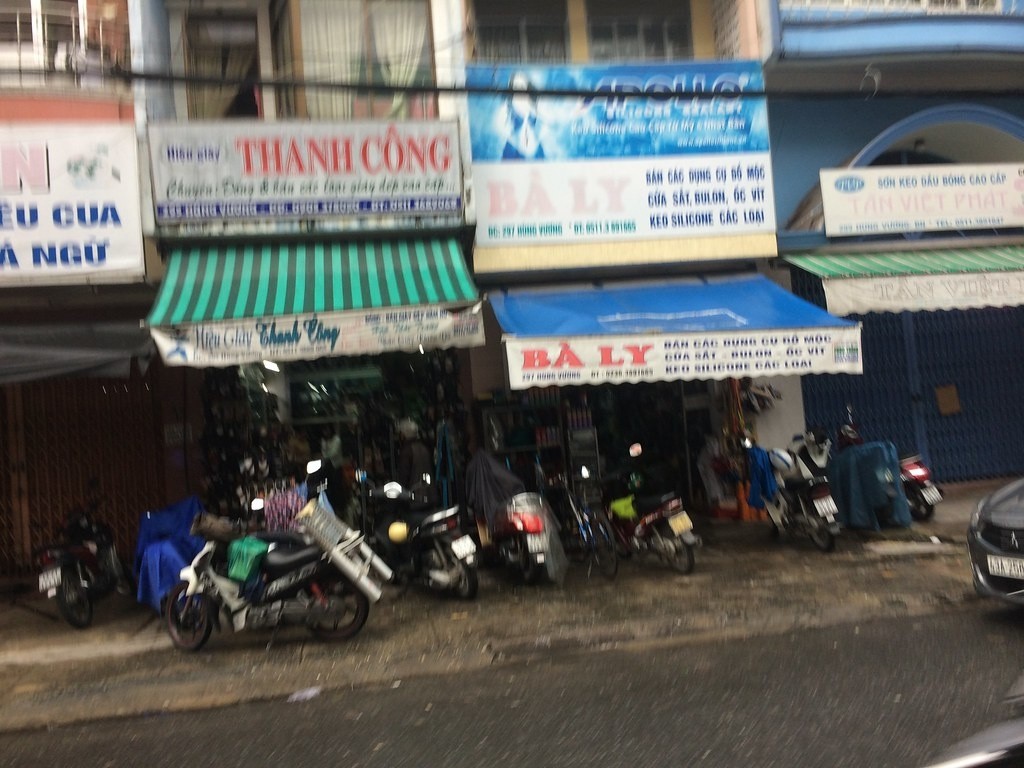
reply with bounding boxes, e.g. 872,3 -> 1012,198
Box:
489,278 -> 864,390
148,237 -> 486,367
783,245 -> 1024,317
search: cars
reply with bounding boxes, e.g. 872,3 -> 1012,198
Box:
967,478 -> 1024,605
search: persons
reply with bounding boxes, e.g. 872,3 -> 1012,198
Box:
393,422 -> 433,490
499,71 -> 543,159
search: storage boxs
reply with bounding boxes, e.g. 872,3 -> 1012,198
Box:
226,537 -> 269,582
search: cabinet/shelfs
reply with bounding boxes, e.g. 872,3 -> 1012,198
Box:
481,404 -> 605,554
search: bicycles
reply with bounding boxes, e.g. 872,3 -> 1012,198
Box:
543,465 -> 618,582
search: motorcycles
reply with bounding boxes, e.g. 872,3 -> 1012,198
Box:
251,459 -> 480,603
792,405 -> 948,528
28,476 -> 136,631
744,435 -> 840,551
580,442 -> 704,577
463,451 -> 555,583
166,498 -> 369,654
